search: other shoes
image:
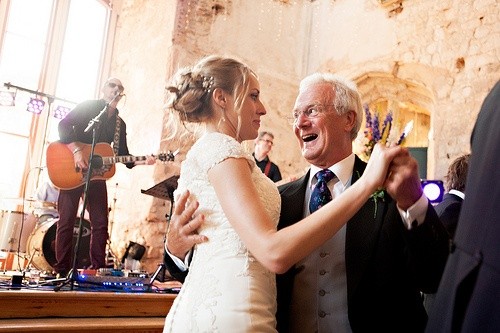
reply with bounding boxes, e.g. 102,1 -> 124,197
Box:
57,274 -> 67,279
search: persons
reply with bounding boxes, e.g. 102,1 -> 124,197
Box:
421,78 -> 500,333
251,131 -> 282,183
164,72 -> 450,333
53,76 -> 156,279
163,55 -> 409,333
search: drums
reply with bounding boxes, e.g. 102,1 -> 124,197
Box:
0,210 -> 38,253
28,216 -> 92,271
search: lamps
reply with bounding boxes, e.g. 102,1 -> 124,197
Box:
0,82 -> 16,107
54,101 -> 71,119
26,89 -> 45,114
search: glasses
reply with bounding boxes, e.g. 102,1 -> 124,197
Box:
107,83 -> 124,91
260,139 -> 273,144
287,104 -> 335,125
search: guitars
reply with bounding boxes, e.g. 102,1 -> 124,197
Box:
43,139 -> 174,191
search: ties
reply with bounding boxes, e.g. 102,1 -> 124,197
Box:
309,169 -> 336,215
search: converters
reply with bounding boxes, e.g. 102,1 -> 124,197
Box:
10,275 -> 23,286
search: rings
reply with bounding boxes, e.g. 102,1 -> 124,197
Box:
386,170 -> 392,179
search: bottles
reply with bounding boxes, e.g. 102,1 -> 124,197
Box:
97,268 -> 113,275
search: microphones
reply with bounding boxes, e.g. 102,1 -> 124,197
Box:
113,91 -> 125,96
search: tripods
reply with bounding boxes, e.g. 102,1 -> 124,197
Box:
141,175 -> 182,287
42,96 -> 124,293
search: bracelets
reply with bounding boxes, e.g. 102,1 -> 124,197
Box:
73,147 -> 80,153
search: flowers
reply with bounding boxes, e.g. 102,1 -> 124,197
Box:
363,103 -> 414,162
